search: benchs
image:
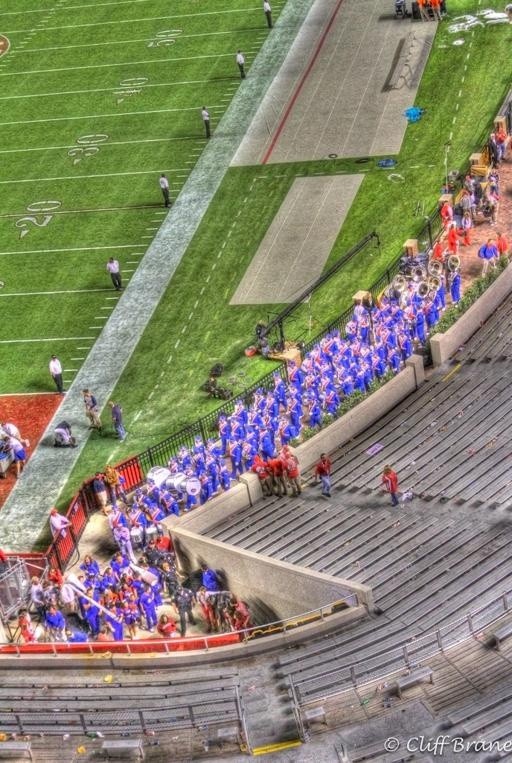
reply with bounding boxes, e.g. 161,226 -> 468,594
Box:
0,283 -> 511,762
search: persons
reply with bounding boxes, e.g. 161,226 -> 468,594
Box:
202,106 -> 210,139
428,0 -> 443,22
0,125 -> 508,645
264,0 -> 273,28
236,50 -> 246,78
417,0 -> 433,22
